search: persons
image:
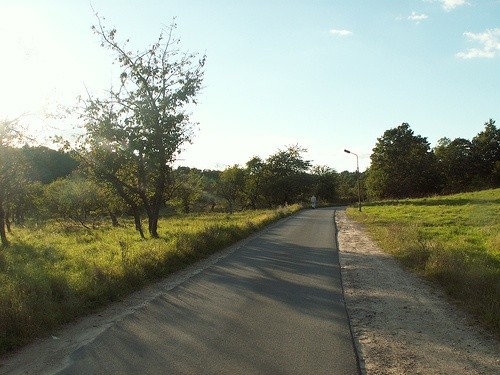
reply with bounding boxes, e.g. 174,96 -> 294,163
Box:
311,195 -> 316,209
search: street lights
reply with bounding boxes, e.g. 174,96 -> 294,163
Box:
344,149 -> 361,213
